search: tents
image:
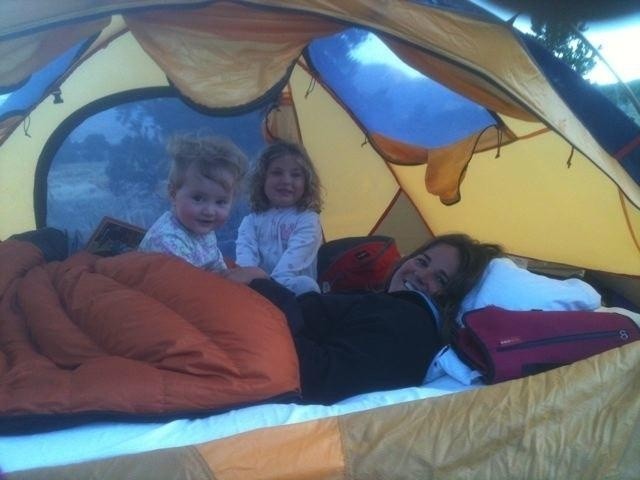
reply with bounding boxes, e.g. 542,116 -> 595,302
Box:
0,0 -> 639,479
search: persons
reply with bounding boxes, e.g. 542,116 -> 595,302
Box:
135,128 -> 228,277
201,230 -> 507,406
233,140 -> 324,298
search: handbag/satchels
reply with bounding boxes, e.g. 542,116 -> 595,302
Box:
448,305 -> 640,384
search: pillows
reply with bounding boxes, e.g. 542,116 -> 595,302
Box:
424,258 -> 603,385
